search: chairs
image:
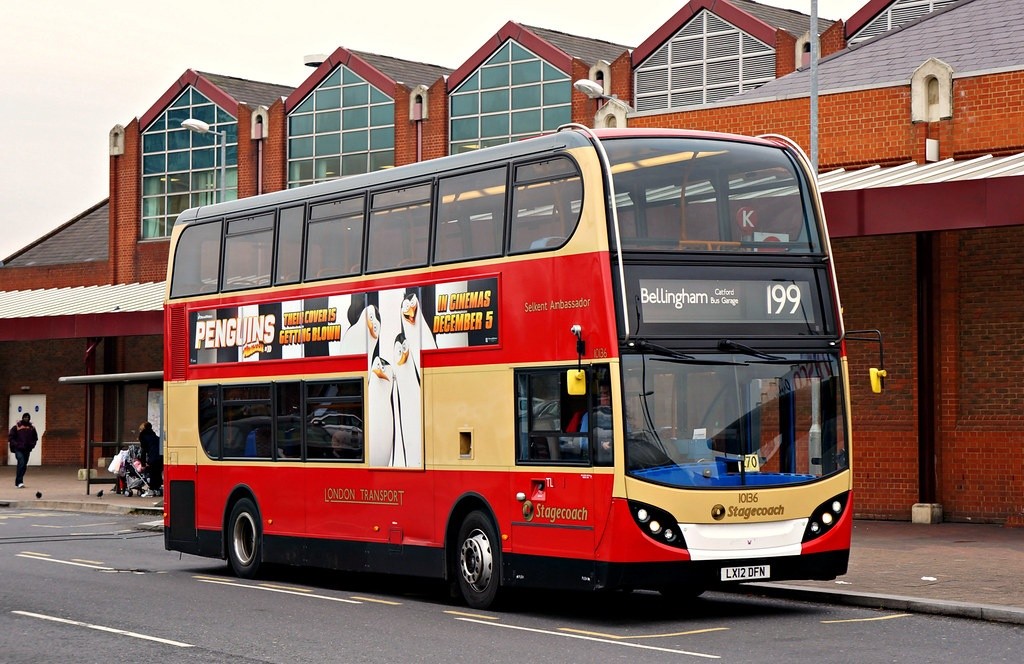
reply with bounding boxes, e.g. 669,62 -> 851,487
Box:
198,259 -> 424,294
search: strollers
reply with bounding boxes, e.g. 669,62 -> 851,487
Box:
106,445 -> 162,497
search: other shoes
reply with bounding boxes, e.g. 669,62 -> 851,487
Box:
15,485 -> 19,488
154,490 -> 161,496
141,489 -> 156,499
18,483 -> 24,488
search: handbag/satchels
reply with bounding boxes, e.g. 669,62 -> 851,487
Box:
9,441 -> 17,453
114,462 -> 127,477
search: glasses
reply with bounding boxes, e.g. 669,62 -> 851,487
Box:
601,391 -> 611,396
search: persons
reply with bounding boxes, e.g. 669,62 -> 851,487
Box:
138,422 -> 163,497
579,381 -> 633,452
7,412 -> 38,488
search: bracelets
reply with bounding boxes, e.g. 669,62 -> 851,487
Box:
601,440 -> 604,447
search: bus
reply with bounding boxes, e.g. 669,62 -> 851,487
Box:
161,121 -> 888,610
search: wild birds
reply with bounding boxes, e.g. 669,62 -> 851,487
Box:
97,490 -> 103,499
36,492 -> 42,499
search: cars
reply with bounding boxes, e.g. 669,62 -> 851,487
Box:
513,391 -> 614,466
199,411 -> 364,459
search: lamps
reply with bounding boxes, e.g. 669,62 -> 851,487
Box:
572,77 -> 617,99
